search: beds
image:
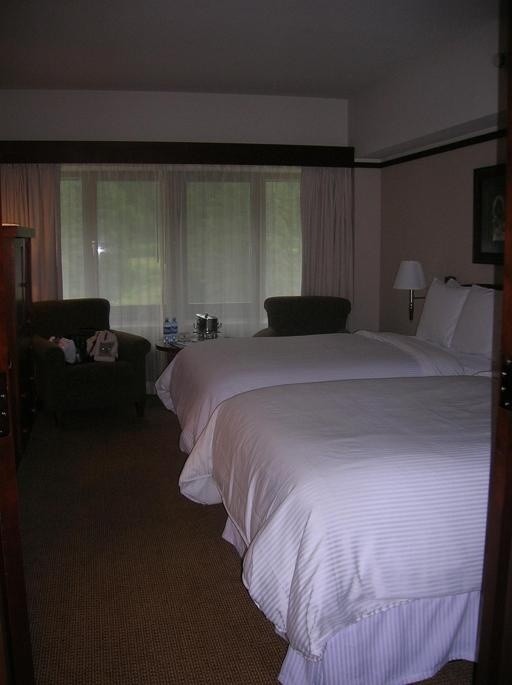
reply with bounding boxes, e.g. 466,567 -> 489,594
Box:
179,372 -> 493,685
155,276 -> 504,456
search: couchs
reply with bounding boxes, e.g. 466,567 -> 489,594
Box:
252,296 -> 351,337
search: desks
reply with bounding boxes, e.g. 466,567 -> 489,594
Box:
156,334 -> 229,355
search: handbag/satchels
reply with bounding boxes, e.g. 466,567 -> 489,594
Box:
49,335 -> 82,364
85,329 -> 120,362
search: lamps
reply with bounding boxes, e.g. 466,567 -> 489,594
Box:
392,261 -> 429,323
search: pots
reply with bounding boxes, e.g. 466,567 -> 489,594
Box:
193,312 -> 222,332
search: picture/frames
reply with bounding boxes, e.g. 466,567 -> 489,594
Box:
471,165 -> 505,265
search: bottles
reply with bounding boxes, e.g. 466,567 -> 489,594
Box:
163,317 -> 177,344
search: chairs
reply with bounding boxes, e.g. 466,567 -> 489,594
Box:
27,297 -> 152,429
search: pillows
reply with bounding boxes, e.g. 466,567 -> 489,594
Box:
418,277 -> 496,361
